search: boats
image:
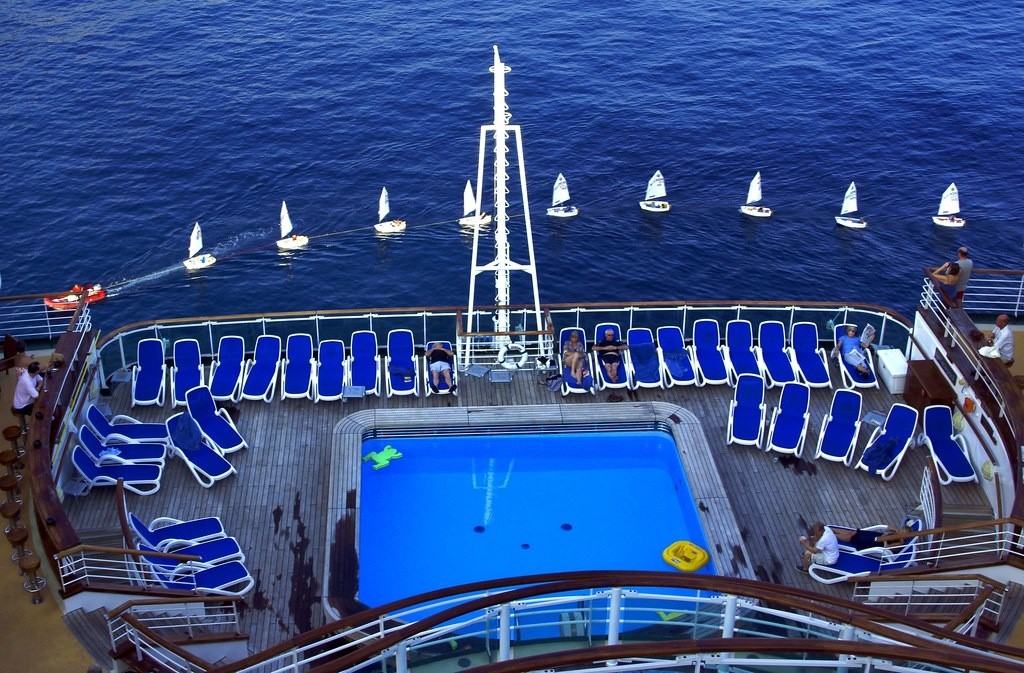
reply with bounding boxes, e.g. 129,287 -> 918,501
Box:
43,283 -> 107,311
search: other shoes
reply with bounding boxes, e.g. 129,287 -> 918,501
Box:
432,385 -> 438,393
608,394 -> 623,402
801,553 -> 810,562
449,385 -> 456,392
795,565 -> 808,574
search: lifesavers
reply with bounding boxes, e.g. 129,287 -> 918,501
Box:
498,343 -> 528,370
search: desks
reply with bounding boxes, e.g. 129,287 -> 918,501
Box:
874,347 -> 908,395
903,360 -> 956,428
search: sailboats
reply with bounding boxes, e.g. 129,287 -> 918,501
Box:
932,182 -> 966,228
835,180 -> 867,229
740,171 -> 772,218
545,172 -> 579,218
457,179 -> 493,229
373,186 -> 407,236
638,170 -> 671,212
182,221 -> 216,270
275,200 -> 309,251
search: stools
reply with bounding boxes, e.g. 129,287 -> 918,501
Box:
0,405 -> 47,604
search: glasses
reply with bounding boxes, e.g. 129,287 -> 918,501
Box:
607,334 -> 613,336
852,330 -> 856,333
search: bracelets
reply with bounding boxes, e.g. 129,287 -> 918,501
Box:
989,341 -> 991,343
617,346 -> 618,349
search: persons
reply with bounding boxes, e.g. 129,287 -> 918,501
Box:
563,331 -> 586,385
73,285 -> 84,298
988,314 -> 1014,364
945,246 -> 973,307
932,262 -> 962,307
832,527 -> 913,548
835,326 -> 875,375
795,519 -> 839,573
14,361 -> 43,416
591,328 -> 628,382
424,343 -> 457,393
15,342 -> 33,379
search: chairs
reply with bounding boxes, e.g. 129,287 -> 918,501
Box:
74,324 -> 455,602
558,319 -> 981,584
